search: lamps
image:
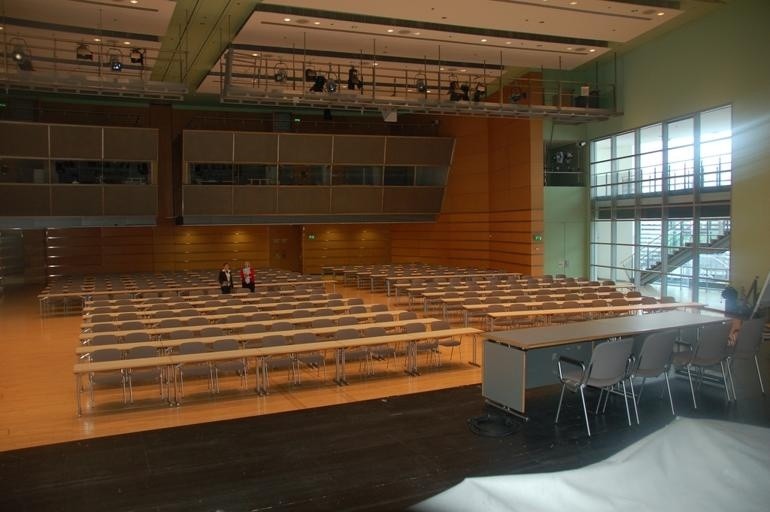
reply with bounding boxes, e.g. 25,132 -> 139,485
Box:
274,60 -> 426,92
11,43 -> 143,71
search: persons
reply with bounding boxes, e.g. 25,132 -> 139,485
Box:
347,65 -> 361,89
218,262 -> 233,293
449,81 -> 459,100
240,259 -> 256,291
474,86 -> 484,101
460,82 -> 471,101
